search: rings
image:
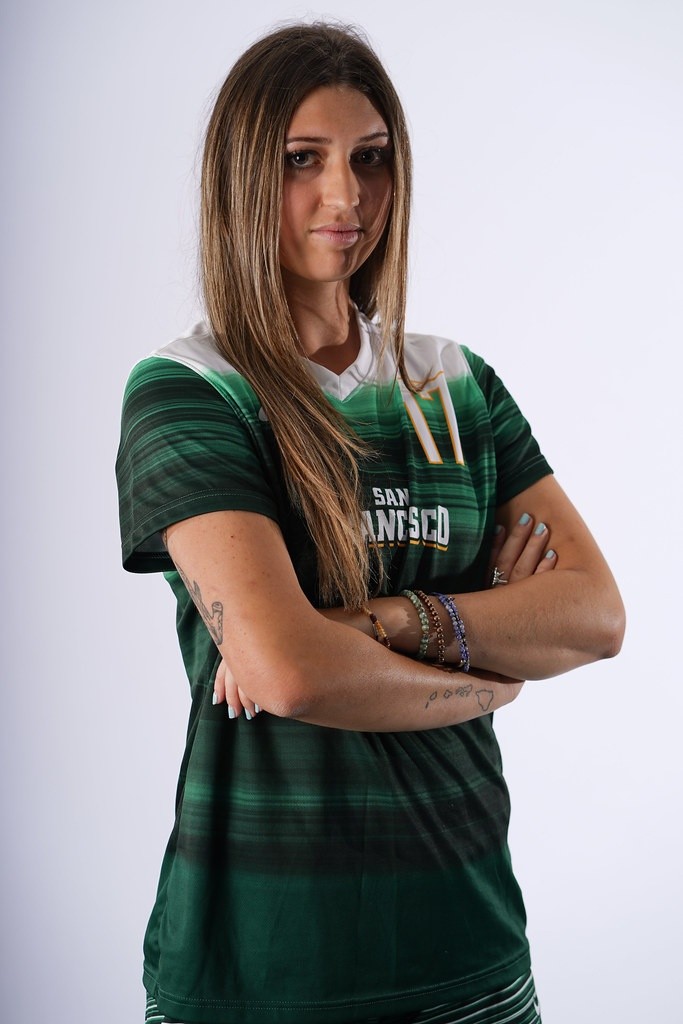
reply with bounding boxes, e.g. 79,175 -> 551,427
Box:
490,566 -> 509,588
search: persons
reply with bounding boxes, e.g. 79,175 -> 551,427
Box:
116,16 -> 627,1024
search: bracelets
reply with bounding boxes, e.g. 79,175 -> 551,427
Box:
361,603 -> 390,648
414,590 -> 445,667
431,591 -> 469,672
399,590 -> 431,663
